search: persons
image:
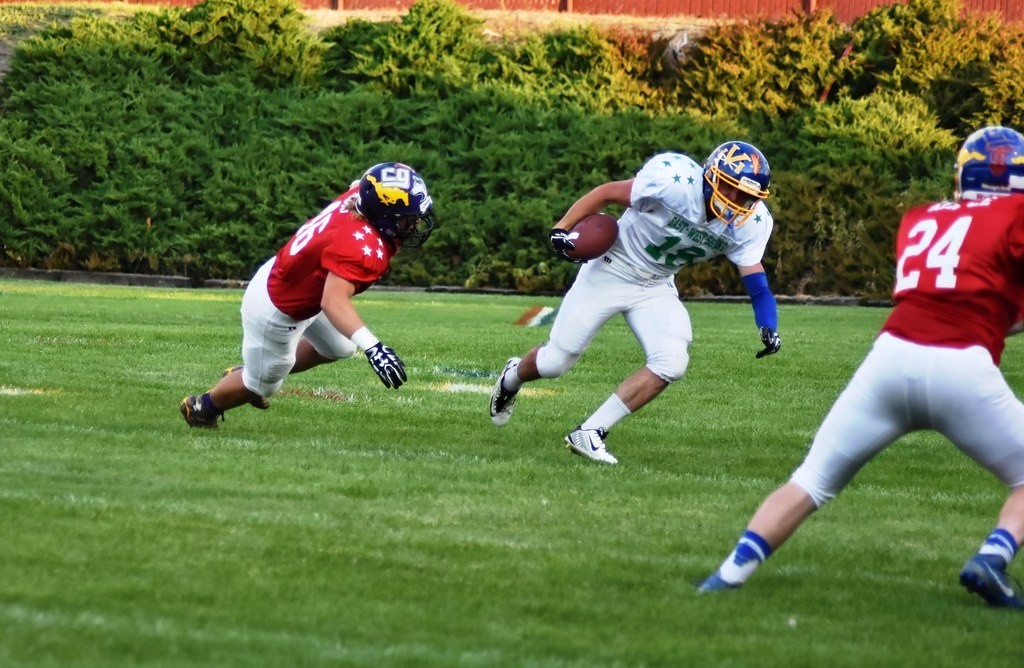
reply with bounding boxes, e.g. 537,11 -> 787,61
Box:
489,140 -> 781,464
702,127 -> 1024,610
181,163 -> 435,430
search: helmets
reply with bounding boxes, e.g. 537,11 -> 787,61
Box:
703,141 -> 771,200
356,161 -> 433,238
958,125 -> 1024,195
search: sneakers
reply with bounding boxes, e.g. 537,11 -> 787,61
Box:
223,366 -> 270,409
179,390 -> 225,430
490,357 -> 521,426
698,573 -> 742,593
959,554 -> 1024,609
564,425 -> 618,464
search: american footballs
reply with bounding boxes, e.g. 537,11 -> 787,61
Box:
564,211 -> 619,261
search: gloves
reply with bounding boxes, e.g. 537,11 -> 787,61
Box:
548,228 -> 584,263
364,342 -> 407,390
756,326 -> 781,359
